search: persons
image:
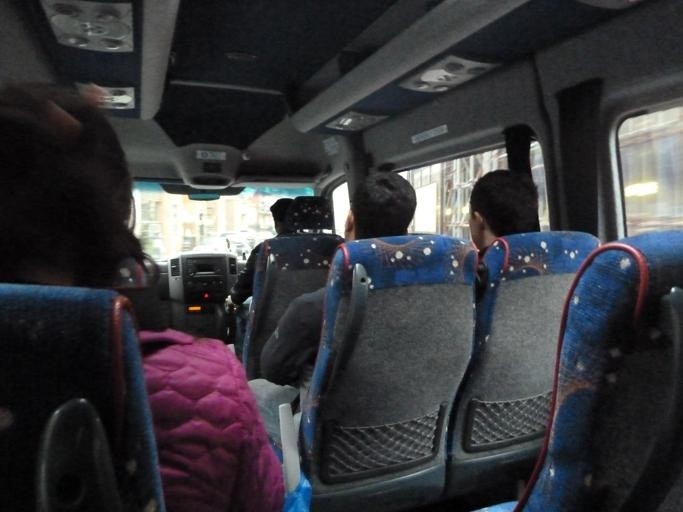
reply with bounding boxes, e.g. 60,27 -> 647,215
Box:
254,170 -> 420,385
219,197 -> 297,356
0,76 -> 288,510
462,167 -> 542,264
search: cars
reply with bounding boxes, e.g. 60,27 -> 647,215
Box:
194,228 -> 275,259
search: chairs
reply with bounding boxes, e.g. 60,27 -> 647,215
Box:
242,200 -> 347,382
444,228 -> 612,511
514,231 -> 683,511
0,285 -> 170,512
300,234 -> 483,512
